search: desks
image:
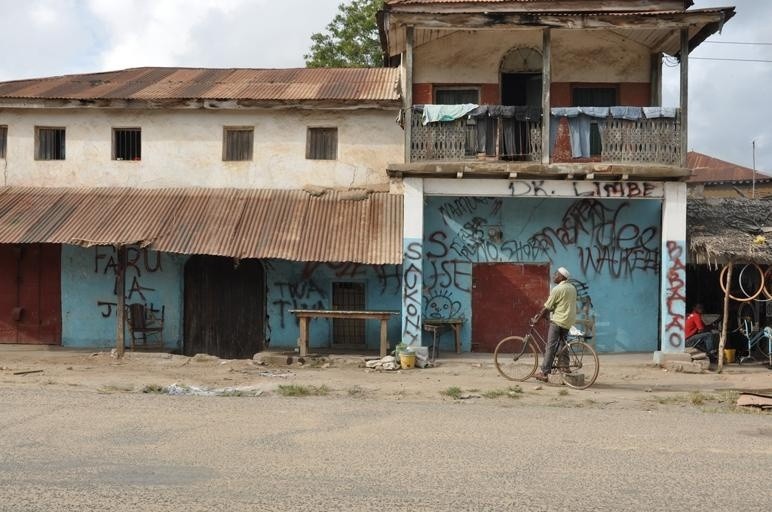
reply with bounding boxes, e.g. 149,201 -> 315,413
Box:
287,309 -> 400,359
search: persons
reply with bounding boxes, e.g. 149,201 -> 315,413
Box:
531,266 -> 578,383
685,301 -> 718,360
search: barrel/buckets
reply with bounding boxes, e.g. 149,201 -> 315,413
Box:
399,351 -> 417,369
725,348 -> 736,363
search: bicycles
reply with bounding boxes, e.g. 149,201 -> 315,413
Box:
494,305 -> 600,391
732,300 -> 771,371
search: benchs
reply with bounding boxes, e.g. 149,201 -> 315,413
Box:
423,319 -> 462,353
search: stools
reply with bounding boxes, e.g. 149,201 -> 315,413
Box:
422,324 -> 442,363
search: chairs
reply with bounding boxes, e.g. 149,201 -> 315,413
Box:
128,303 -> 164,351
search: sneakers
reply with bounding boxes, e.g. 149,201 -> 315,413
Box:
535,373 -> 549,383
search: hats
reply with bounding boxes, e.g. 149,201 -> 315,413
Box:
558,266 -> 570,279
